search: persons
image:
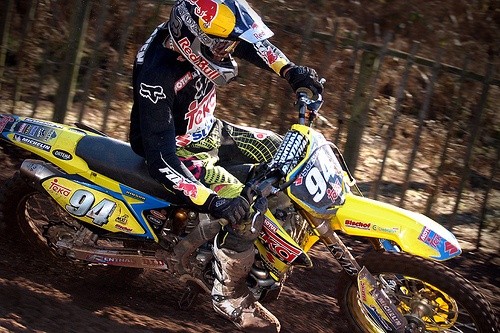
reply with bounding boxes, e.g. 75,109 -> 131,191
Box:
129,0 -> 323,333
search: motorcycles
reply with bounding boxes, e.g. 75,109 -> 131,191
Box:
0,78 -> 499,332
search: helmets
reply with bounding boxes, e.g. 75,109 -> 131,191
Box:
167,0 -> 274,86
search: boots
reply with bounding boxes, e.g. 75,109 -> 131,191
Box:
210,234 -> 283,333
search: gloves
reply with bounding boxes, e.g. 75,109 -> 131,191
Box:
208,194 -> 252,230
284,65 -> 325,95
